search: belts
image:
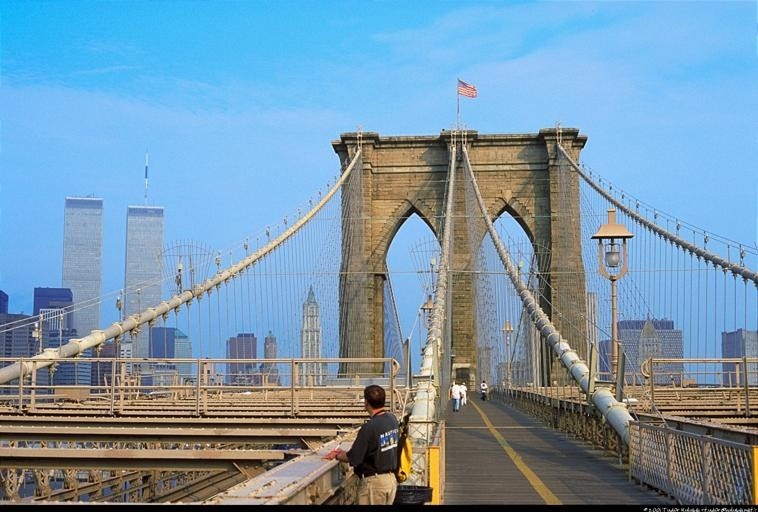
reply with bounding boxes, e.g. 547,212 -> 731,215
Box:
357,470 -> 395,480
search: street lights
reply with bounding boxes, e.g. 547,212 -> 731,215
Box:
502,319 -> 514,389
590,210 -> 635,398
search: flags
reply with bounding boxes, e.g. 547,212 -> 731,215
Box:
458,79 -> 477,100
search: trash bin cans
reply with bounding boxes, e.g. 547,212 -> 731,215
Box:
392,485 -> 433,506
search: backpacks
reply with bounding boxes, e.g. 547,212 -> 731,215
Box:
396,425 -> 413,483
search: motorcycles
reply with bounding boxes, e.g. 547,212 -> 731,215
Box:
479,388 -> 488,401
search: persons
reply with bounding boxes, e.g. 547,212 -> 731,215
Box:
320,383 -> 401,505
480,380 -> 489,401
335,454 -> 338,459
451,381 -> 463,411
460,381 -> 468,406
449,384 -> 453,396
